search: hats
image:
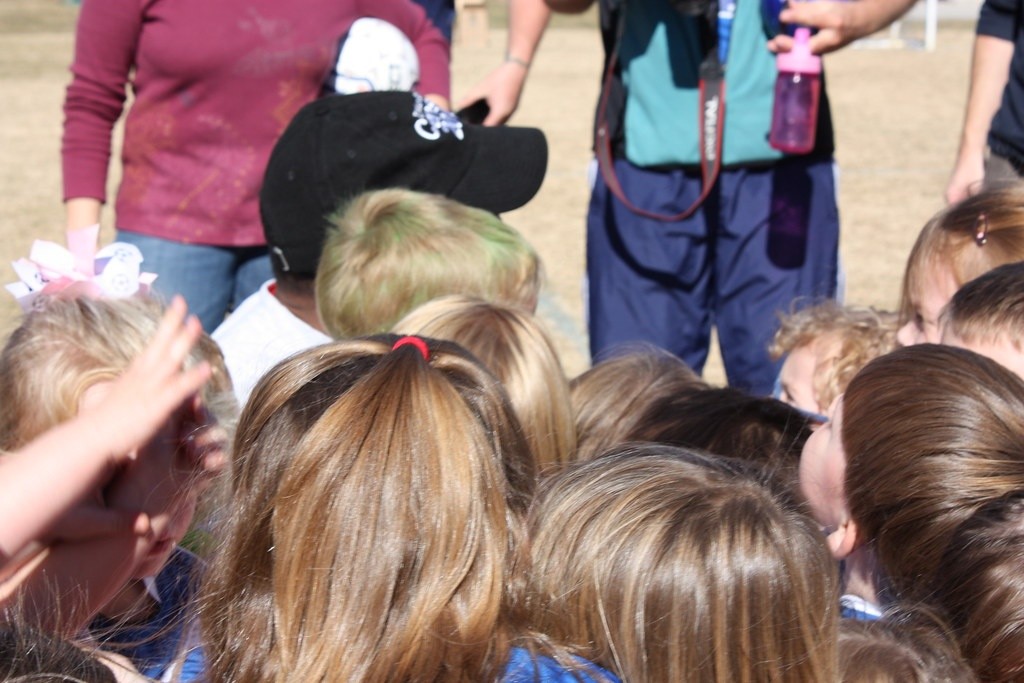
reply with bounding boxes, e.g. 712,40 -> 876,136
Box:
259,89 -> 553,279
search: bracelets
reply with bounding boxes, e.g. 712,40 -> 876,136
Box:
505,53 -> 530,70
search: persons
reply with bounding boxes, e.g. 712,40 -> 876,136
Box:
947,0 -> 1024,213
1,92 -> 1023,683
470,0 -> 921,400
60,0 -> 451,338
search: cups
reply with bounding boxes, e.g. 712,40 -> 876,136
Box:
768,28 -> 823,153
